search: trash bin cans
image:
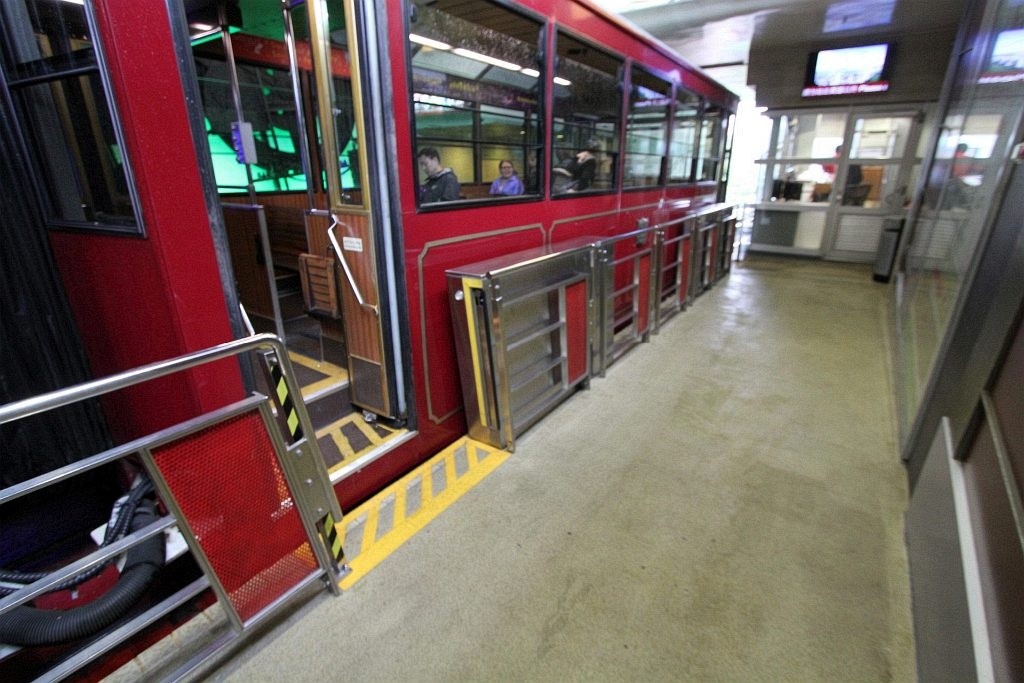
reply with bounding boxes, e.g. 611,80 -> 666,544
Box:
872,219 -> 905,284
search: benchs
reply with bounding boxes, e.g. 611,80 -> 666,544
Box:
254,205 -> 309,255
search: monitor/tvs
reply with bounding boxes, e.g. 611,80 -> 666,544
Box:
799,41 -> 893,100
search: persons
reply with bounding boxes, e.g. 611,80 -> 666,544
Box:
418,148 -> 459,203
551,141 -> 602,195
949,143 -> 973,177
488,160 -> 525,196
823,144 -> 842,174
